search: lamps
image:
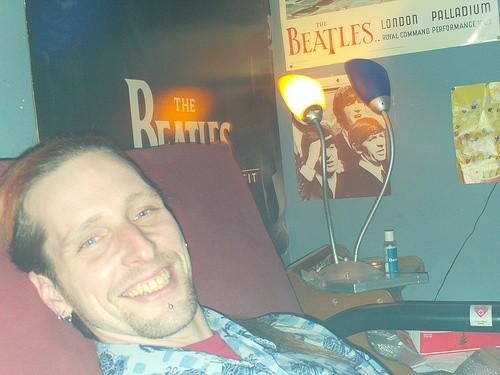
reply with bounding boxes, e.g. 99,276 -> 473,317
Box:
275,59 -> 395,282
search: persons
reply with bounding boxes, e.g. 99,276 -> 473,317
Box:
347,117 -> 392,197
327,84 -> 369,180
296,126 -> 354,200
0,133 -> 393,375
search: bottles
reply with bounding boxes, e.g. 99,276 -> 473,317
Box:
384,230 -> 399,280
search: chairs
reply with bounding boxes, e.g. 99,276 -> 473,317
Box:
0,142 -> 500,374
